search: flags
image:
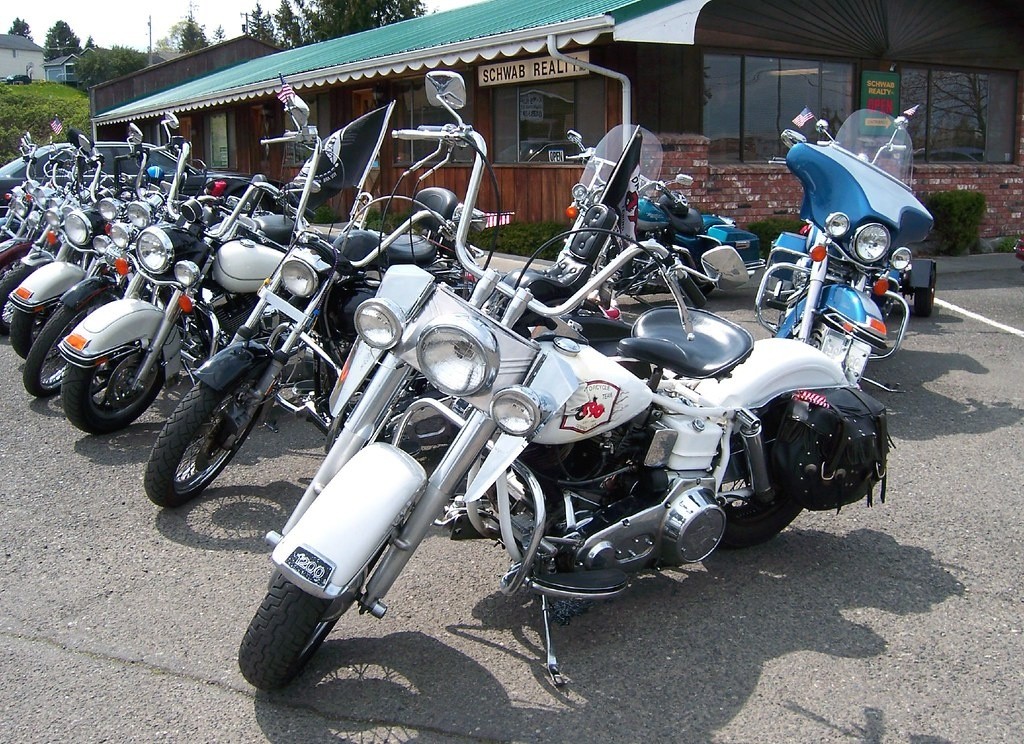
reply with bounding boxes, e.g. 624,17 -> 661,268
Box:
797,219 -> 828,254
483,212 -> 515,229
792,105 -> 815,129
49,114 -> 64,135
276,71 -> 310,127
282,101 -> 396,222
599,125 -> 644,254
898,102 -> 923,119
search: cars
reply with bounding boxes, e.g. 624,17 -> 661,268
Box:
498,138 -> 586,163
0,140 -> 299,234
929,146 -> 984,162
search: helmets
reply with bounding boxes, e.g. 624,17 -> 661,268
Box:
659,191 -> 689,214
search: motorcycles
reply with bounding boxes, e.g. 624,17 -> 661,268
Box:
0,71 -> 894,691
755,116 -> 938,393
557,123 -> 759,307
